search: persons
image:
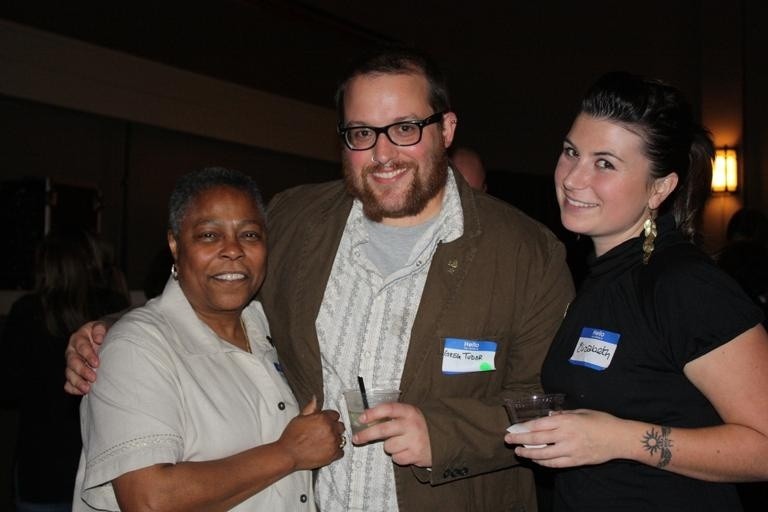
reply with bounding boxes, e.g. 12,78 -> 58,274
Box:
447,144 -> 486,196
62,53 -> 575,510
504,73 -> 768,509
709,208 -> 768,267
714,238 -> 768,330
74,167 -> 345,512
0,225 -> 133,512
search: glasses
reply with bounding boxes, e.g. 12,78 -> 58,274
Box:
342,114 -> 443,154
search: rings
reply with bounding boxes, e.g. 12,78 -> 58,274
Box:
339,434 -> 344,448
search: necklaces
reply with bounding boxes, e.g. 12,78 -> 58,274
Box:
238,316 -> 251,353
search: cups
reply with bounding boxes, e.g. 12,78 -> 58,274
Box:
340,388 -> 402,445
501,392 -> 571,428
